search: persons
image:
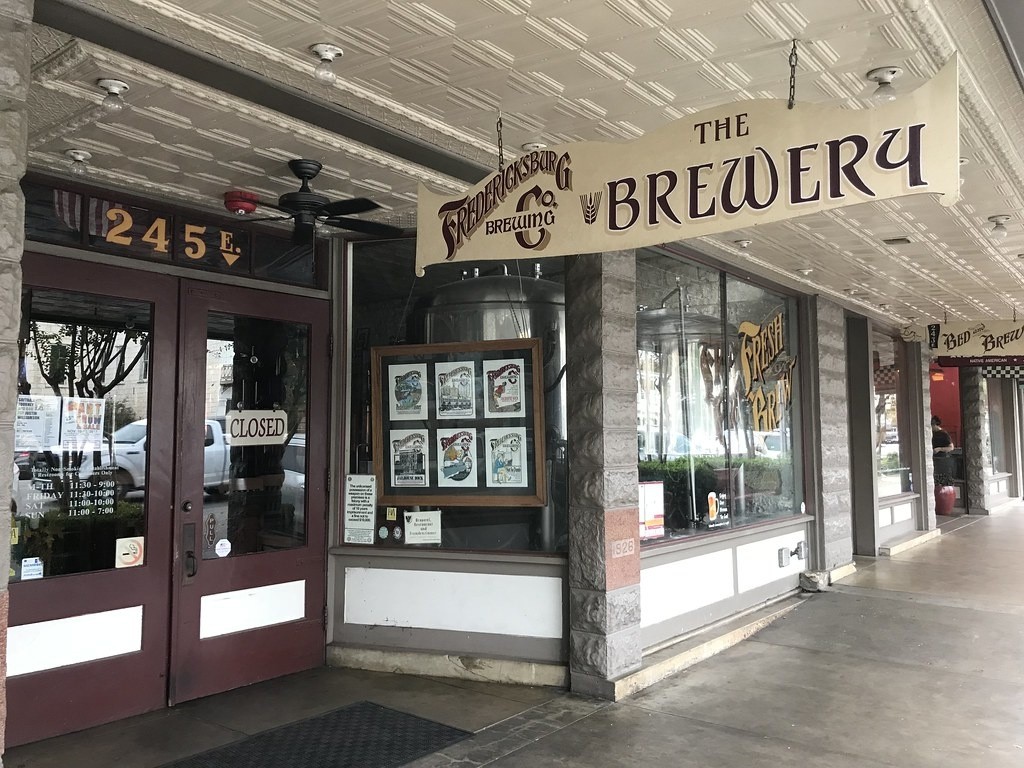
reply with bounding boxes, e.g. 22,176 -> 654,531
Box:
930,415 -> 955,474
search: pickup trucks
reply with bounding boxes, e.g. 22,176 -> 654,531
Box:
30,416 -> 231,503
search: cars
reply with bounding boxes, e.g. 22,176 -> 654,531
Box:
760,432 -> 789,459
280,434 -> 306,536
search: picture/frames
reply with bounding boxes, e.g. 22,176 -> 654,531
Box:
483,359 -> 525,419
485,426 -> 528,487
435,361 -> 476,420
390,429 -> 429,487
389,363 -> 427,421
437,428 -> 478,487
